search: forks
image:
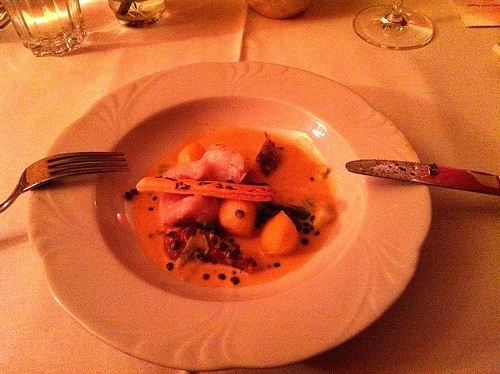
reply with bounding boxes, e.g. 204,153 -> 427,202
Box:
0,152 -> 129,213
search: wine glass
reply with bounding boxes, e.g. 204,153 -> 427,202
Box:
353,0 -> 435,50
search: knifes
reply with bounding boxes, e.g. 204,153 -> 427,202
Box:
345,159 -> 500,198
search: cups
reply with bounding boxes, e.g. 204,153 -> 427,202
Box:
2,0 -> 87,58
245,0 -> 309,20
108,0 -> 166,28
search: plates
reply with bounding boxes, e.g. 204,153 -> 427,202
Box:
22,61 -> 432,369
